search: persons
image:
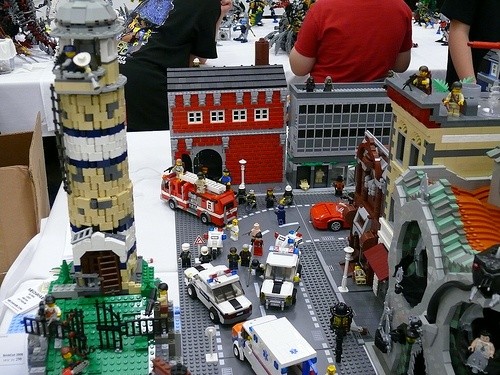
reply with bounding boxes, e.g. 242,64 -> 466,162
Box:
314,169 -> 325,182
466,332 -> 495,373
288,0 -> 413,83
304,76 -> 316,92
60,346 -> 83,370
54,43 -> 93,75
274,204 -> 287,225
439,0 -> 500,91
156,283 -> 169,317
323,75 -> 335,92
117,0 -> 232,131
412,65 -> 433,96
43,296 -> 61,322
178,219 -> 265,279
172,158 -> 294,209
443,80 -> 465,118
334,175 -> 345,197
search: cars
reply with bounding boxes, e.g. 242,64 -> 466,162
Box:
311,201 -> 350,231
185,260 -> 252,325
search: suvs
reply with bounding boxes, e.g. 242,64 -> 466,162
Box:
261,226 -> 304,309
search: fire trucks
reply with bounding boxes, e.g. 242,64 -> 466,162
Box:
160,165 -> 238,226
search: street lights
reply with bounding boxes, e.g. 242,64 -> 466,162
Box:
331,304 -> 352,364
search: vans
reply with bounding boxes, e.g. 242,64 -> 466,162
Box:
231,315 -> 318,375
